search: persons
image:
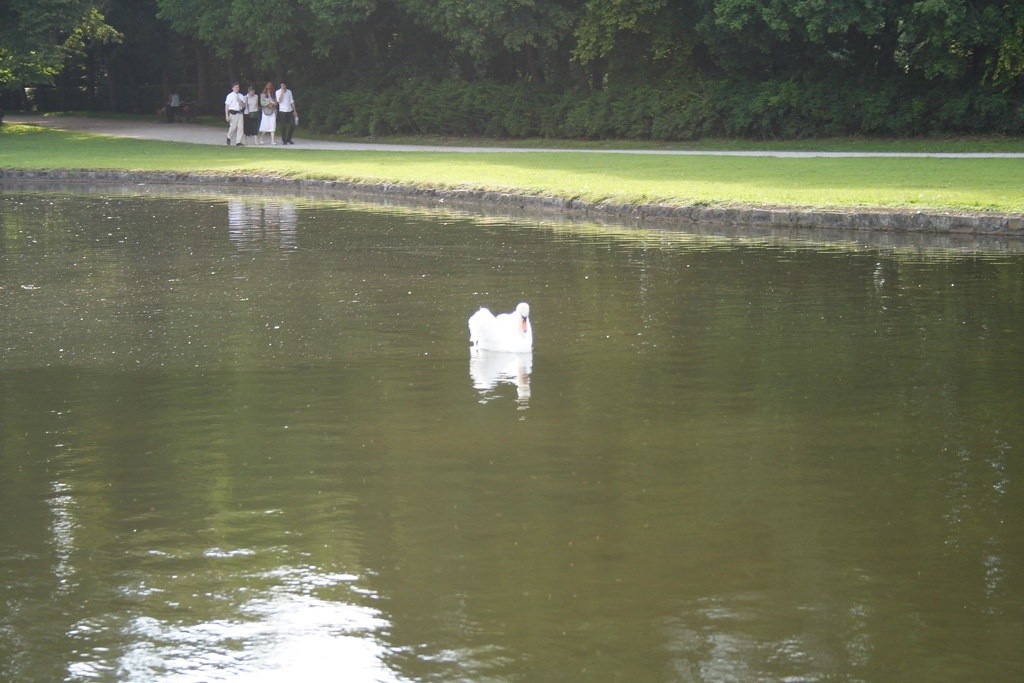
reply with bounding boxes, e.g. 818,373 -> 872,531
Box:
223,81 -> 245,146
243,85 -> 260,146
157,87 -> 184,123
258,80 -> 278,145
274,81 -> 299,144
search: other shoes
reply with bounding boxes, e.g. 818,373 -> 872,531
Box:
259,141 -> 263,145
271,142 -> 276,145
227,138 -> 230,145
283,142 -> 287,145
236,143 -> 244,146
289,140 -> 294,144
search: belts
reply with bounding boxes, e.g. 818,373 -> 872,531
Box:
236,111 -> 243,113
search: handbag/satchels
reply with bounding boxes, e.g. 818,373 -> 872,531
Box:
294,116 -> 299,125
248,112 -> 259,118
264,108 -> 272,115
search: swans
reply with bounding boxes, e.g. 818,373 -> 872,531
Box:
467,301 -> 536,354
